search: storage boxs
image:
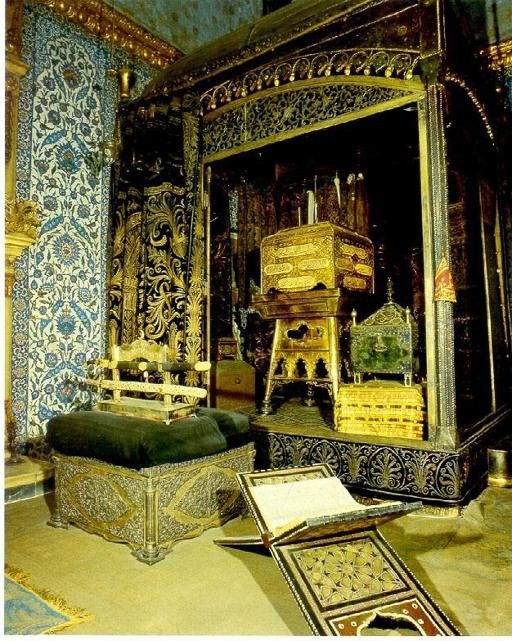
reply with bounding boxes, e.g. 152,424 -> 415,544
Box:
259,221 -> 377,292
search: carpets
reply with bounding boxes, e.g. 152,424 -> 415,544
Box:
4,560 -> 94,634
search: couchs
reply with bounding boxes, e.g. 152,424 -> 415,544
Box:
47,405 -> 256,564
